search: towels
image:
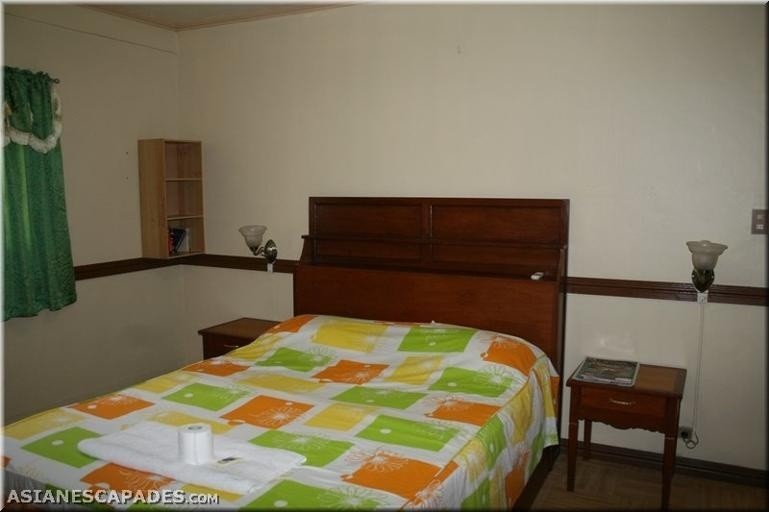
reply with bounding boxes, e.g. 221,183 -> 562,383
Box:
75,417 -> 314,496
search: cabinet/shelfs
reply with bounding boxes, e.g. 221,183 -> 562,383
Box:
137,137 -> 206,261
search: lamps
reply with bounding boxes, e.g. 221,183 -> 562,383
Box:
685,240 -> 730,294
238,224 -> 279,265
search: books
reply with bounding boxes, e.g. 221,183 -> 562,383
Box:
176,226 -> 190,252
570,355 -> 641,388
168,228 -> 187,254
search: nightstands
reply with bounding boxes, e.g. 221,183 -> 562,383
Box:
198,317 -> 283,361
562,355 -> 688,512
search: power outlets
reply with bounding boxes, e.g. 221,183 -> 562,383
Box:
677,426 -> 694,441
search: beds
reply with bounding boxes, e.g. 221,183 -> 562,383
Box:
3,197 -> 572,509
